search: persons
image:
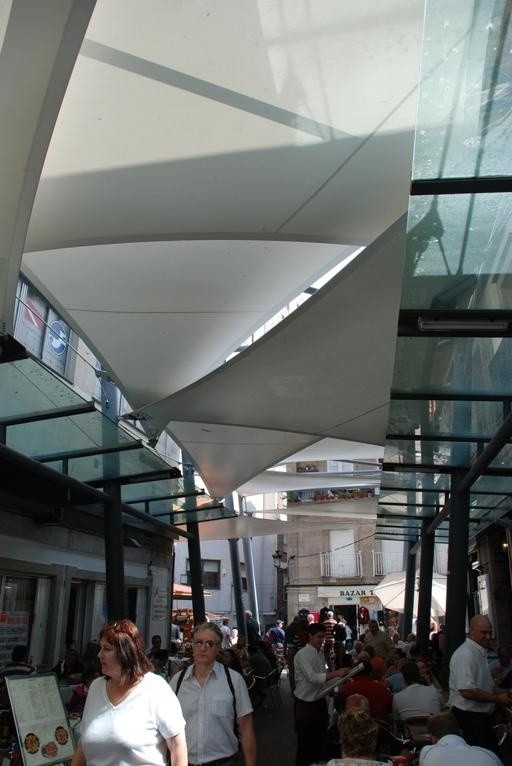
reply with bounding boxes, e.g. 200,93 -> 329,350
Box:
66,665 -> 101,718
70,620 -> 189,766
169,621 -> 258,766
146,610 -> 285,689
2,644 -> 36,705
285,606 -> 510,766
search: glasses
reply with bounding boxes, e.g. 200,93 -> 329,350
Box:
191,638 -> 221,649
106,620 -> 133,638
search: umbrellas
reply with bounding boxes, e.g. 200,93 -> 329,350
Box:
371,564 -> 449,617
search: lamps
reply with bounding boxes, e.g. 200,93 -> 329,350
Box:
272,550 -> 289,569
